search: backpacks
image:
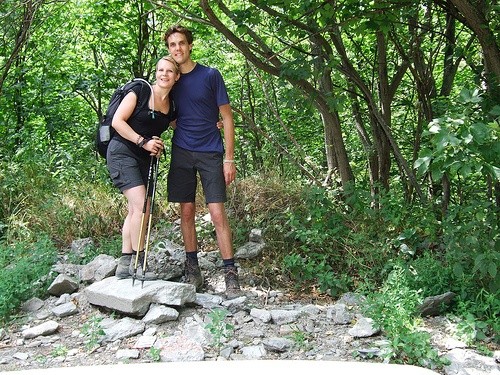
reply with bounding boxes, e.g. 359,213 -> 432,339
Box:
95,78 -> 177,160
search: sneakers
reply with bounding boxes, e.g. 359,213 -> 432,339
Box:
129,251 -> 157,280
115,255 -> 156,278
178,258 -> 202,289
224,266 -> 242,299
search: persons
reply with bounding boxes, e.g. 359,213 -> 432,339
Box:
164,25 -> 241,299
107,56 -> 224,280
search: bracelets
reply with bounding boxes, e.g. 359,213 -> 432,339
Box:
224,160 -> 234,163
